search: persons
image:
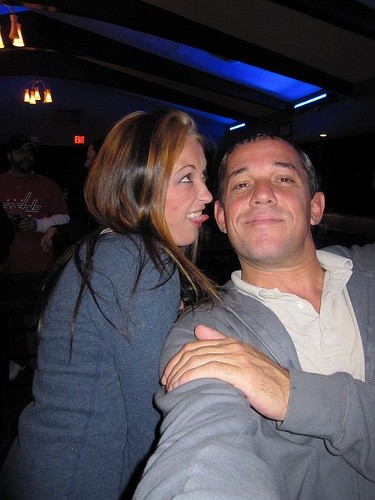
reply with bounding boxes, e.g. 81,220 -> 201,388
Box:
132,133 -> 375,500
0,107 -> 214,500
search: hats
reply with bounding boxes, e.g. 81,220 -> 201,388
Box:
5,134 -> 39,151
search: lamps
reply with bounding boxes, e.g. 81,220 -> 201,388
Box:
0,1 -> 25,49
23,79 -> 54,105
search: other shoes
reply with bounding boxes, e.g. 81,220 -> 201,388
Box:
8,360 -> 27,381
